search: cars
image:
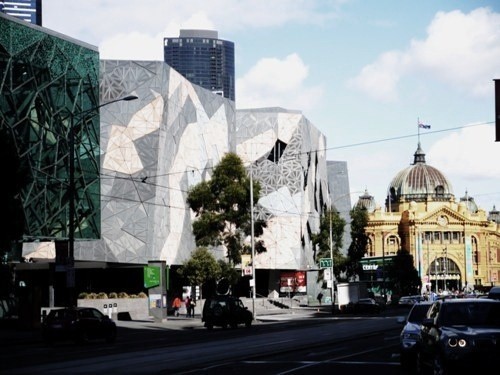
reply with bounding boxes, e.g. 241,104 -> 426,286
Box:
40,307 -> 118,347
202,293 -> 254,331
397,286 -> 500,375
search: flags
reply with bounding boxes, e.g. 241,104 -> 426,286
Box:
418,120 -> 430,129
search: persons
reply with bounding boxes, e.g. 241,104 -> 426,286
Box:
171,297 -> 182,317
185,295 -> 196,318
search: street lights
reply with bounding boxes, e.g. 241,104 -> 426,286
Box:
60,95 -> 139,307
328,191 -> 367,315
248,138 -> 287,323
